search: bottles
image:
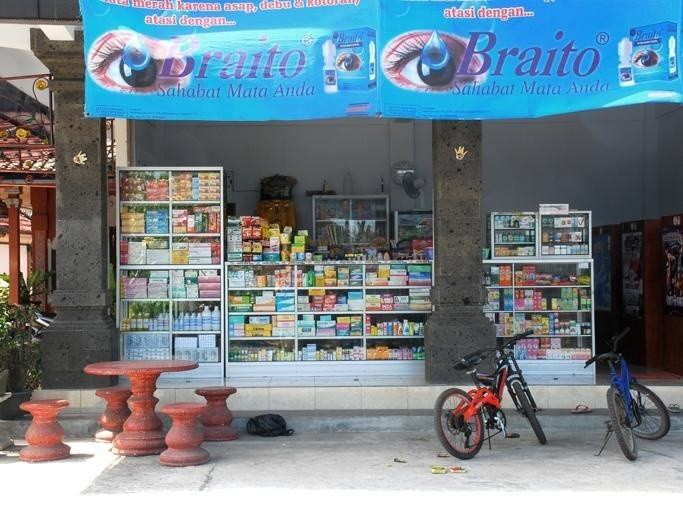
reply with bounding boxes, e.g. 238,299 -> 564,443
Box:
137,308 -> 142,331
189,313 -> 196,331
196,313 -> 203,330
153,318 -> 157,330
184,313 -> 189,330
202,306 -> 212,330
179,312 -> 184,331
157,313 -> 163,330
142,308 -> 150,331
149,317 -> 152,331
130,308 -> 137,331
163,312 -> 169,331
211,306 -> 221,331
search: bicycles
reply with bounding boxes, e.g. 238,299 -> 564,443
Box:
433,328 -> 548,461
584,316 -> 671,463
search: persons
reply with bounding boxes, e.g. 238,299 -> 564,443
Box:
379,30 -> 487,94
86,31 -> 189,93
632,49 -> 660,68
335,52 -> 362,72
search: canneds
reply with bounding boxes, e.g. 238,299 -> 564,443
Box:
126,319 -> 131,331
122,319 -> 126,331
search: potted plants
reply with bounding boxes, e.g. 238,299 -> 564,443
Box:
1,301 -> 44,419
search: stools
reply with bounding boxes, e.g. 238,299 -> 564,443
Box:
17,399 -> 71,461
94,386 -> 133,442
195,386 -> 241,442
160,401 -> 210,466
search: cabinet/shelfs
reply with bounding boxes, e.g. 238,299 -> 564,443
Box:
539,210 -> 593,260
115,166 -> 224,379
486,210 -> 538,260
224,261 -> 598,376
310,194 -> 390,255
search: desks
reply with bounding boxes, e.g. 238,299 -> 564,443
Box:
84,359 -> 198,455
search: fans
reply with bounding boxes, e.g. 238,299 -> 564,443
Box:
400,171 -> 432,214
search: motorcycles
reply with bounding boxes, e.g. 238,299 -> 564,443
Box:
25,302 -> 56,339
